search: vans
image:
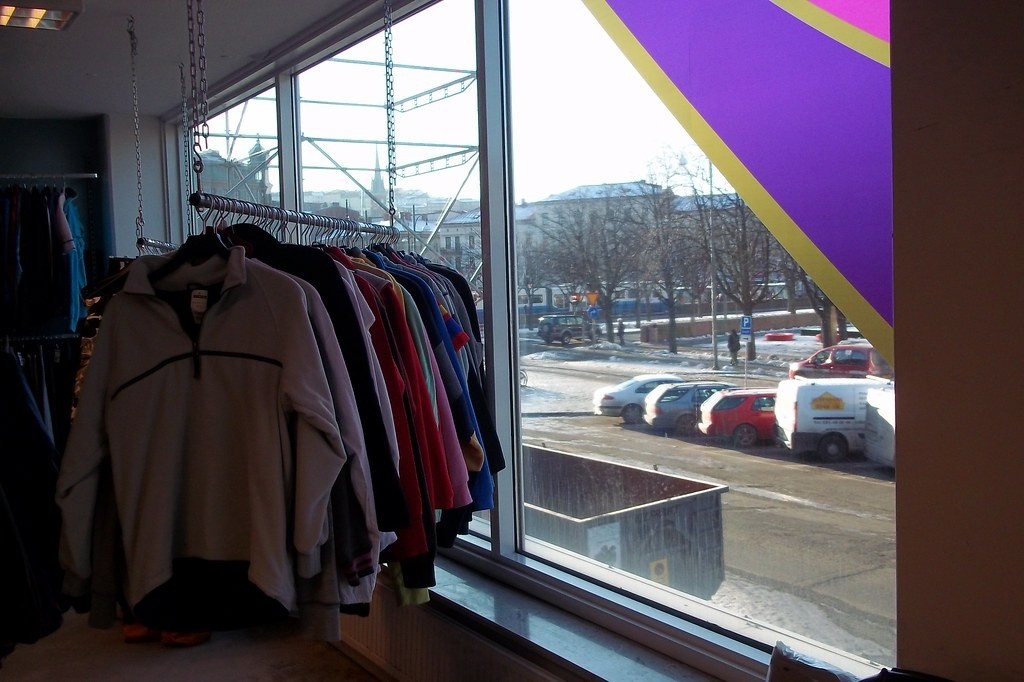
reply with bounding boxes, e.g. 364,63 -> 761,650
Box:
771,375 -> 894,465
861,382 -> 895,473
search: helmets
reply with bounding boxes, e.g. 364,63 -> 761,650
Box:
617,318 -> 623,322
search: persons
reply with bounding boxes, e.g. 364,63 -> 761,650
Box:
728,329 -> 740,366
616,318 -> 625,345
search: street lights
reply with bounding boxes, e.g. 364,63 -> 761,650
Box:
411,204 -> 467,252
678,141 -> 721,371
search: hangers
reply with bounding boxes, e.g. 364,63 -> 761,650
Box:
148,192 -> 404,284
0,333 -> 82,354
0,171 -> 68,198
79,237 -> 181,300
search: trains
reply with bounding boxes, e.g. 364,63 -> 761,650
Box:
469,279 -> 828,327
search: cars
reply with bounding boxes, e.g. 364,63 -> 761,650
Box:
787,338 -> 890,381
641,378 -> 744,436
593,371 -> 689,424
694,385 -> 778,448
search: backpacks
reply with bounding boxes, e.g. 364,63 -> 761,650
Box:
731,335 -> 741,351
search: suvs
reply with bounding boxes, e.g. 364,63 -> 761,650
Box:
536,313 -> 603,345
